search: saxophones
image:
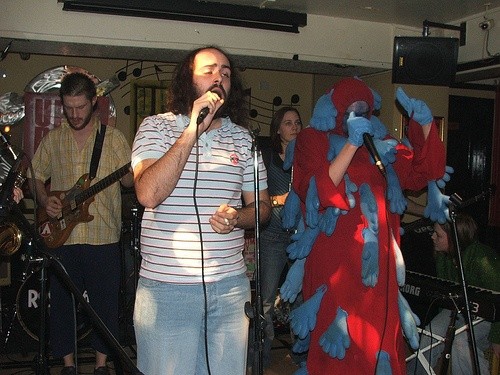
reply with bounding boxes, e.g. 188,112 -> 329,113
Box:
0,131 -> 32,261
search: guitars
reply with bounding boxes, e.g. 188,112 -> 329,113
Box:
34,161 -> 132,248
399,184 -> 496,247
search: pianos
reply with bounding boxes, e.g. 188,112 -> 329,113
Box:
398,269 -> 500,324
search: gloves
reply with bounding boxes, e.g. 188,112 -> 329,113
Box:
372,90 -> 381,109
328,134 -> 346,160
280,174 -> 379,303
424,166 -> 453,224
376,351 -> 392,375
284,135 -> 295,174
310,90 -> 337,131
347,112 -> 375,146
320,307 -> 349,360
371,115 -> 407,214
294,362 -> 307,375
289,285 -> 327,353
396,87 -> 433,125
393,236 -> 420,350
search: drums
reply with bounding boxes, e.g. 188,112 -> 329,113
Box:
12,264 -> 94,347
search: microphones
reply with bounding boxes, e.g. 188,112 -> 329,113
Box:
363,133 -> 386,176
197,90 -> 222,124
0,152 -> 24,208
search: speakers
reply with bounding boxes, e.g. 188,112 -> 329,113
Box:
392,36 -> 459,87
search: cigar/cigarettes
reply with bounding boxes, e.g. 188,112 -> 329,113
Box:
225,218 -> 229,226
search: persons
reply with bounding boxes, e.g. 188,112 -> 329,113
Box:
280,76 -> 446,375
13,187 -> 24,204
247,107 -> 303,375
26,73 -> 134,375
403,195 -> 499,375
132,45 -> 271,375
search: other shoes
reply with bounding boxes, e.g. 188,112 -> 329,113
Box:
61,364 -> 110,375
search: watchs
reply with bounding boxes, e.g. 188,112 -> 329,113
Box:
272,195 -> 279,207
